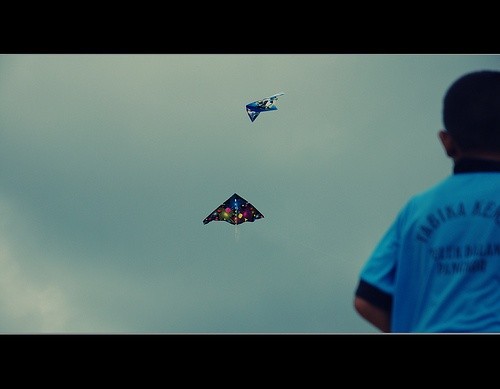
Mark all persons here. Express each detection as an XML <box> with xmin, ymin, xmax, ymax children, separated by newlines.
<box><xmin>352</xmin><ymin>69</ymin><xmax>499</xmax><ymax>333</ymax></box>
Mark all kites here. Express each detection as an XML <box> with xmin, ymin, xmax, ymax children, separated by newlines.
<box><xmin>202</xmin><ymin>192</ymin><xmax>265</xmax><ymax>227</ymax></box>
<box><xmin>244</xmin><ymin>93</ymin><xmax>285</xmax><ymax>123</ymax></box>
